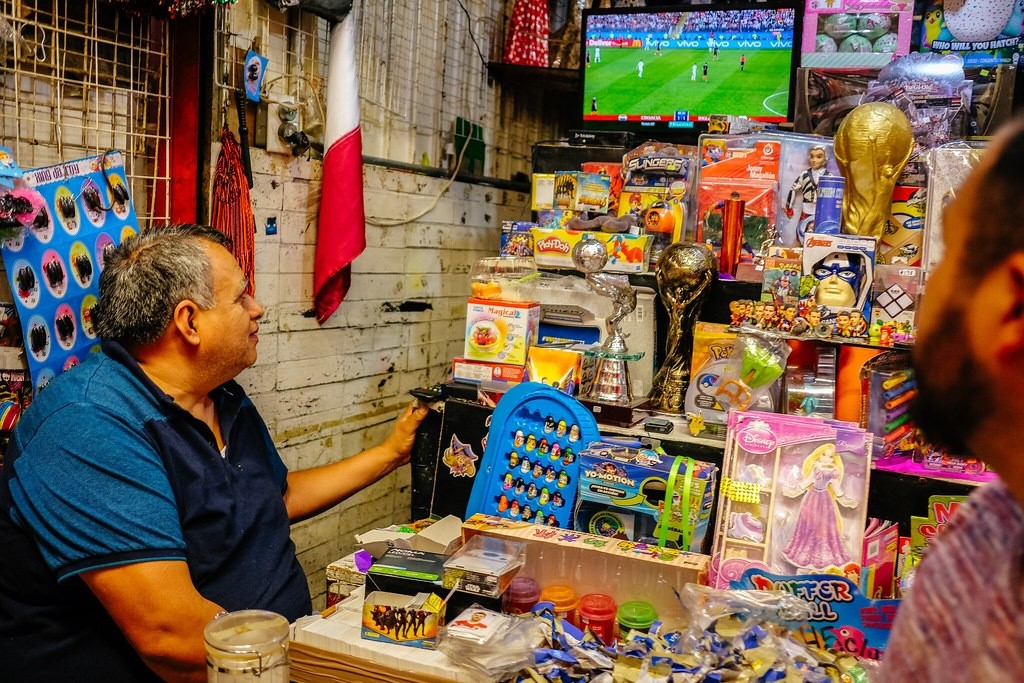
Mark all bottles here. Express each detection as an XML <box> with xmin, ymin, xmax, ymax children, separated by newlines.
<box><xmin>720</xmin><ymin>192</ymin><xmax>745</xmax><ymax>278</ymax></box>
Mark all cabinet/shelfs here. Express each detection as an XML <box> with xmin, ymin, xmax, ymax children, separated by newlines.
<box><xmin>410</xmin><ymin>257</ymin><xmax>998</xmax><ymax>547</ymax></box>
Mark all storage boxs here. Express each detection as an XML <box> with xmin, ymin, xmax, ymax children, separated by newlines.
<box><xmin>452</xmin><ymin>357</ymin><xmax>525</xmax><ymax>385</ymax></box>
<box><xmin>800</xmin><ymin>0</ymin><xmax>915</xmax><ymax>67</ymax></box>
<box><xmin>803</xmin><ymin>229</ymin><xmax>876</xmax><ymax>336</ymax></box>
<box><xmin>532</xmin><ymin>172</ymin><xmax>610</xmax><ymax>211</ymax></box>
<box><xmin>289</xmin><ymin>513</ymin><xmax>711</xmax><ymax>683</ymax></box>
<box><xmin>552</xmin><ymin>169</ymin><xmax>612</xmax><ymax>214</ymax></box>
<box><xmin>465</xmin><ymin>298</ymin><xmax>540</xmax><ymax>364</ymax></box>
<box><xmin>573</xmin><ymin>438</ymin><xmax>719</xmax><ymax>555</ymax></box>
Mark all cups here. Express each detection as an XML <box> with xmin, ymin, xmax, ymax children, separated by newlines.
<box><xmin>203</xmin><ymin>609</ymin><xmax>289</xmax><ymax>683</ymax></box>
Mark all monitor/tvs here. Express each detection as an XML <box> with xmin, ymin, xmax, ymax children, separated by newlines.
<box><xmin>580</xmin><ymin>0</ymin><xmax>805</xmax><ymax>145</ymax></box>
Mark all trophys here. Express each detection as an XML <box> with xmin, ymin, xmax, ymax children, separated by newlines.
<box><xmin>647</xmin><ymin>242</ymin><xmax>717</xmax><ymax>413</ymax></box>
<box><xmin>572</xmin><ymin>239</ymin><xmax>641</xmax><ymax>406</ymax></box>
<box><xmin>831</xmin><ymin>101</ymin><xmax>915</xmax><ymax>267</ymax></box>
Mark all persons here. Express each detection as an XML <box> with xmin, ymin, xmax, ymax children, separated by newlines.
<box><xmin>813</xmin><ymin>252</ymin><xmax>867</xmax><ymax>307</ymax></box>
<box><xmin>729</xmin><ymin>299</ymin><xmax>917</xmax><ymax>347</ymax></box>
<box><xmin>0</xmin><ymin>223</ymin><xmax>428</xmax><ymax>683</ymax></box>
<box><xmin>772</xmin><ymin>275</ymin><xmax>791</xmax><ymax>307</ymax></box>
<box><xmin>861</xmin><ymin>117</ymin><xmax>1024</xmax><ymax>682</ymax></box>
<box><xmin>780</xmin><ymin>143</ymin><xmax>834</xmax><ymax>248</ymax></box>
<box><xmin>582</xmin><ymin>8</ymin><xmax>795</xmax><ymax>116</ymax></box>
